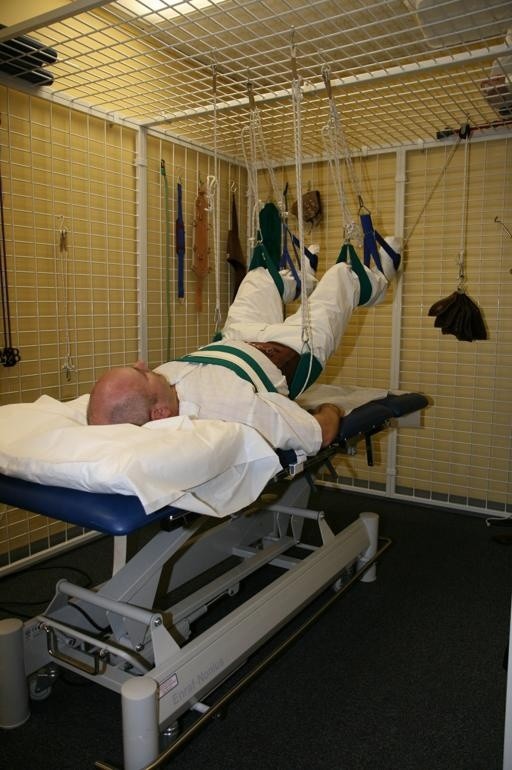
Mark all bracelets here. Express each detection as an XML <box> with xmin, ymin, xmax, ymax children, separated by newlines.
<box><xmin>318</xmin><ymin>403</ymin><xmax>345</xmax><ymax>418</ymax></box>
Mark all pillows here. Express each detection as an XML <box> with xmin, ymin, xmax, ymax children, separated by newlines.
<box><xmin>0</xmin><ymin>395</ymin><xmax>283</xmax><ymax>519</ymax></box>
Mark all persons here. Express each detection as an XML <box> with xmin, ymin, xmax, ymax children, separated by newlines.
<box><xmin>85</xmin><ymin>234</ymin><xmax>404</xmax><ymax>458</ymax></box>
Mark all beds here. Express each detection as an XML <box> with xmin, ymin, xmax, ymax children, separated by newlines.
<box><xmin>0</xmin><ymin>381</ymin><xmax>432</xmax><ymax>768</ymax></box>
